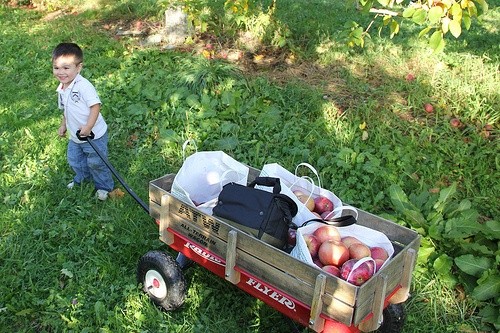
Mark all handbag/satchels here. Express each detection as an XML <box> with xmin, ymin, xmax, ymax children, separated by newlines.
<box><xmin>290</xmin><ymin>206</ymin><xmax>394</xmax><ymax>287</ymax></box>
<box><xmin>170</xmin><ymin>140</ymin><xmax>250</xmax><ymax>216</ymax></box>
<box><xmin>254</xmin><ymin>163</ymin><xmax>343</xmax><ymax>226</ymax></box>
<box><xmin>212</xmin><ymin>176</ymin><xmax>297</xmax><ymax>250</ymax></box>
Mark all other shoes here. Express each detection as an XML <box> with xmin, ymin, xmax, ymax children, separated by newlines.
<box><xmin>67</xmin><ymin>181</ymin><xmax>75</xmax><ymax>189</ymax></box>
<box><xmin>95</xmin><ymin>190</ymin><xmax>108</xmax><ymax>200</ymax></box>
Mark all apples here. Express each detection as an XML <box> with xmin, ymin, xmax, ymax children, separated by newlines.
<box><xmin>287</xmin><ymin>190</ymin><xmax>388</xmax><ymax>285</ymax></box>
<box><xmin>404</xmin><ymin>73</ymin><xmax>492</xmax><ymax>140</ymax></box>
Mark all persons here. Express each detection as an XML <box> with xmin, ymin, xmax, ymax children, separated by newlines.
<box><xmin>51</xmin><ymin>43</ymin><xmax>115</xmax><ymax>201</ymax></box>
